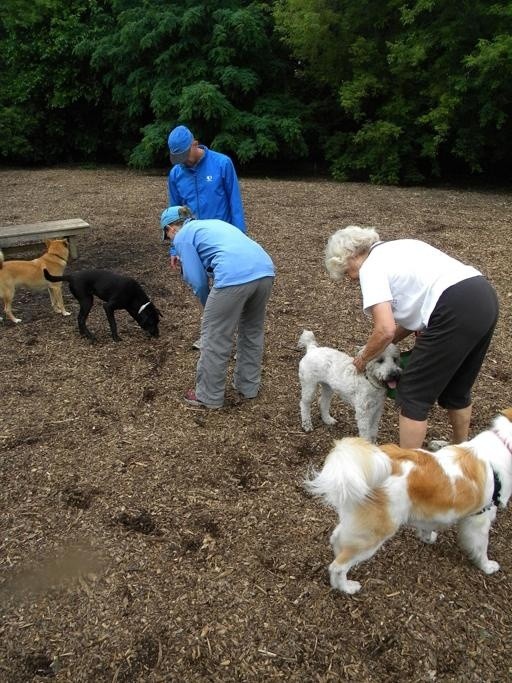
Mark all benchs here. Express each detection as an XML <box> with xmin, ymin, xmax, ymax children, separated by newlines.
<box><xmin>0</xmin><ymin>218</ymin><xmax>91</xmax><ymax>259</ymax></box>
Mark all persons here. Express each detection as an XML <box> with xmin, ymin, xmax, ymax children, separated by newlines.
<box><xmin>159</xmin><ymin>202</ymin><xmax>277</xmax><ymax>409</ymax></box>
<box><xmin>168</xmin><ymin>123</ymin><xmax>247</xmax><ymax>355</ymax></box>
<box><xmin>322</xmin><ymin>223</ymin><xmax>500</xmax><ymax>450</ymax></box>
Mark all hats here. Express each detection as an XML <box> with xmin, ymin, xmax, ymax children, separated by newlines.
<box><xmin>168</xmin><ymin>126</ymin><xmax>193</xmax><ymax>165</ymax></box>
<box><xmin>161</xmin><ymin>206</ymin><xmax>182</xmax><ymax>241</ymax></box>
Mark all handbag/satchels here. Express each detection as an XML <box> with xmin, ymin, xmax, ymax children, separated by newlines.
<box><xmin>387</xmin><ymin>349</ymin><xmax>413</xmax><ymax>399</ymax></box>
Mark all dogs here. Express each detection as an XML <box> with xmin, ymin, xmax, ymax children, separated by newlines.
<box><xmin>0</xmin><ymin>237</ymin><xmax>71</xmax><ymax>323</ymax></box>
<box><xmin>296</xmin><ymin>329</ymin><xmax>403</xmax><ymax>444</ymax></box>
<box><xmin>303</xmin><ymin>405</ymin><xmax>512</xmax><ymax>594</ymax></box>
<box><xmin>43</xmin><ymin>267</ymin><xmax>164</xmax><ymax>342</ymax></box>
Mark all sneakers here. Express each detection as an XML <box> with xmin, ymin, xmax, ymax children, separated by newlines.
<box><xmin>192</xmin><ymin>339</ymin><xmax>201</xmax><ymax>348</ymax></box>
<box><xmin>183</xmin><ymin>388</ymin><xmax>223</xmax><ymax>408</ymax></box>
